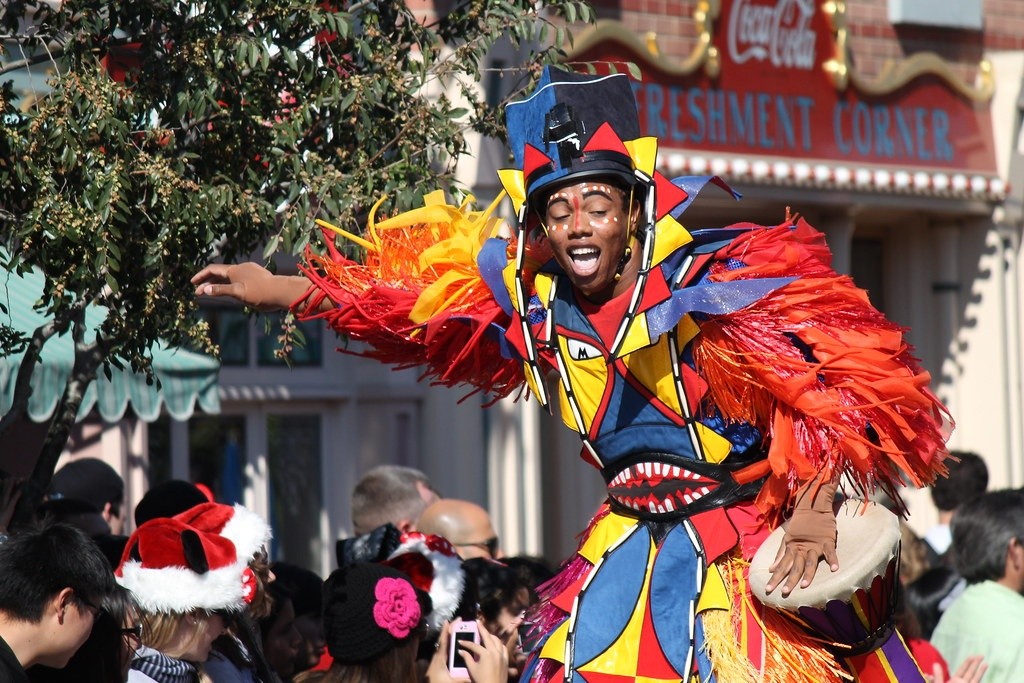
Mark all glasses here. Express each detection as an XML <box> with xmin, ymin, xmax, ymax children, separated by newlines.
<box><xmin>120</xmin><ymin>622</ymin><xmax>144</xmax><ymax>640</ymax></box>
<box><xmin>450</xmin><ymin>536</ymin><xmax>499</xmax><ymax>559</ymax></box>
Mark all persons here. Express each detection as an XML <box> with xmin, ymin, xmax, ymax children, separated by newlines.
<box><xmin>838</xmin><ymin>453</ymin><xmax>1024</xmax><ymax>683</ymax></box>
<box><xmin>0</xmin><ymin>458</ymin><xmax>564</xmax><ymax>683</ymax></box>
<box><xmin>191</xmin><ymin>176</ymin><xmax>950</xmax><ymax>683</ymax></box>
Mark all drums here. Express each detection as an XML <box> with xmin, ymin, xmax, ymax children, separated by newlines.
<box><xmin>747</xmin><ymin>496</ymin><xmax>904</xmax><ymax>658</ymax></box>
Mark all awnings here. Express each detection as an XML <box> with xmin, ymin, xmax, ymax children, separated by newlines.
<box><xmin>0</xmin><ymin>246</ymin><xmax>222</xmax><ymax>420</ymax></box>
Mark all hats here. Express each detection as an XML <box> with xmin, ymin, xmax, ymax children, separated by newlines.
<box><xmin>135</xmin><ymin>479</ymin><xmax>274</xmax><ymax>566</ymax></box>
<box><xmin>318</xmin><ymin>563</ymin><xmax>433</xmax><ymax>664</ymax></box>
<box><xmin>114</xmin><ymin>517</ymin><xmax>257</xmax><ymax>620</ymax></box>
<box><xmin>381</xmin><ymin>531</ymin><xmax>466</xmax><ymax>639</ymax></box>
<box><xmin>336</xmin><ymin>522</ymin><xmax>402</xmax><ymax>567</ymax></box>
<box><xmin>496</xmin><ymin>63</ymin><xmax>649</xmax><ymax>214</ymax></box>
<box><xmin>41</xmin><ymin>458</ymin><xmax>123</xmax><ymax>510</ymax></box>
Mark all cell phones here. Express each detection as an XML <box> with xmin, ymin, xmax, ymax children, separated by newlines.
<box><xmin>449</xmin><ymin>621</ymin><xmax>481</xmax><ymax>678</ymax></box>
